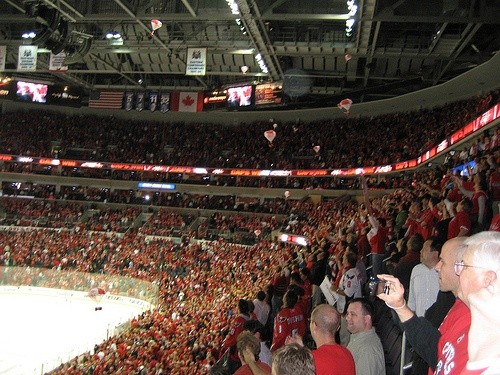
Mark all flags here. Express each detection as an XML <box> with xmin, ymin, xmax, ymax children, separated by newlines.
<box><xmin>170</xmin><ymin>91</ymin><xmax>205</xmax><ymax>113</ymax></box>
<box><xmin>88</xmin><ymin>90</ymin><xmax>124</xmax><ymax>108</ymax></box>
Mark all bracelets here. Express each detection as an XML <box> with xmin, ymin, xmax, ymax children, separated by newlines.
<box><xmin>385</xmin><ymin>299</ymin><xmax>406</xmax><ymax>309</ymax></box>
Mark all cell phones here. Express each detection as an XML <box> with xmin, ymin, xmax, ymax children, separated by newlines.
<box><xmin>292</xmin><ymin>329</ymin><xmax>298</xmax><ymax>338</ymax></box>
<box><xmin>384</xmin><ymin>281</ymin><xmax>390</xmax><ymax>295</ymax></box>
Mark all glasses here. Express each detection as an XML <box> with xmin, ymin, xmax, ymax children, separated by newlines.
<box><xmin>453</xmin><ymin>259</ymin><xmax>484</xmax><ymax>277</ymax></box>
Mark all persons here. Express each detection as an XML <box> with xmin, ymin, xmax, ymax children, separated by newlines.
<box><xmin>0</xmin><ymin>88</ymin><xmax>500</xmax><ymax>375</ymax></box>
